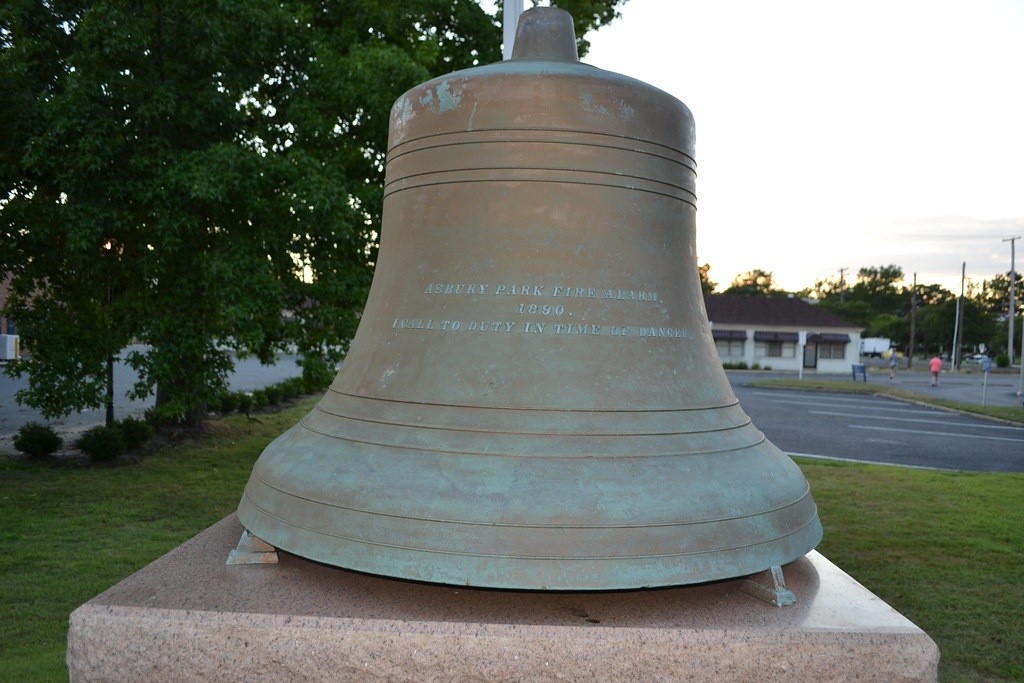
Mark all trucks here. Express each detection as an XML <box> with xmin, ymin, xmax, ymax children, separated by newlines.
<box><xmin>861</xmin><ymin>337</ymin><xmax>892</xmax><ymax>358</ymax></box>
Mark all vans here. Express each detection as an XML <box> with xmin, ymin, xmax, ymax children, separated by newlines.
<box><xmin>965</xmin><ymin>354</ymin><xmax>987</xmax><ymax>364</ymax></box>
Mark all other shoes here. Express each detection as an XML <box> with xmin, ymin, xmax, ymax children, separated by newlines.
<box><xmin>890</xmin><ymin>376</ymin><xmax>893</xmax><ymax>379</ymax></box>
<box><xmin>932</xmin><ymin>384</ymin><xmax>937</xmax><ymax>387</ymax></box>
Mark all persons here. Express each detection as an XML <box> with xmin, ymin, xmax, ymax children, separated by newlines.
<box><xmin>888</xmin><ymin>348</ymin><xmax>899</xmax><ymax>381</ymax></box>
<box><xmin>929</xmin><ymin>353</ymin><xmax>941</xmax><ymax>388</ymax></box>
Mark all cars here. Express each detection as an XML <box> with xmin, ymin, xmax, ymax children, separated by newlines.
<box><xmin>881</xmin><ymin>348</ymin><xmax>903</xmax><ymax>360</ymax></box>
